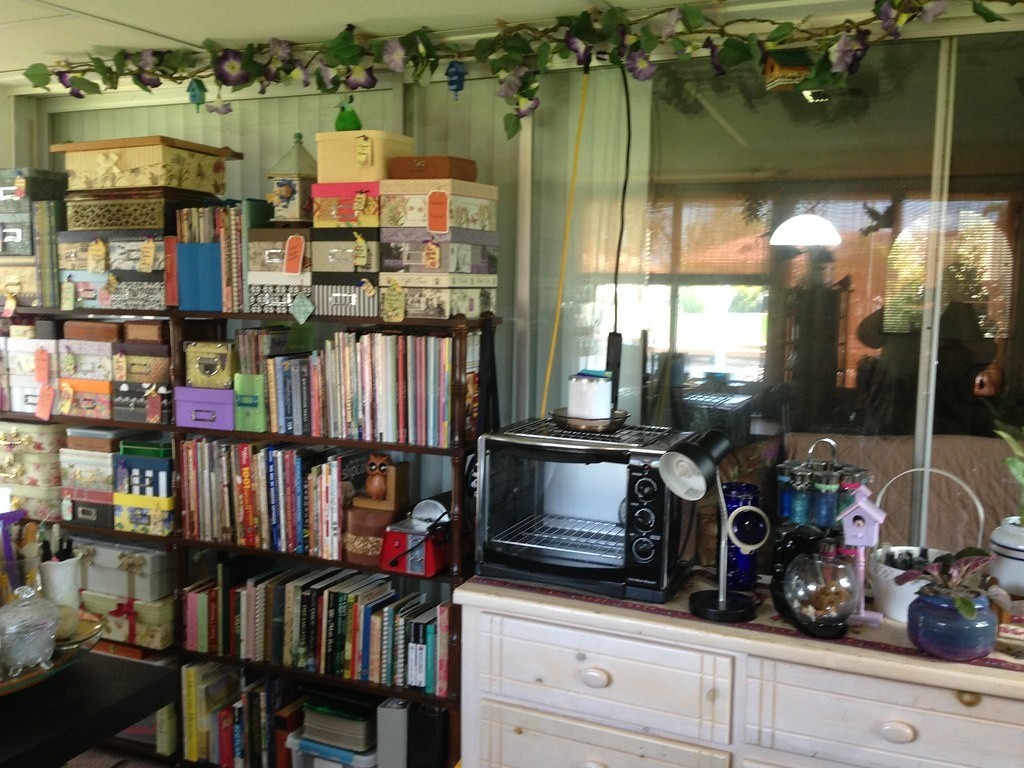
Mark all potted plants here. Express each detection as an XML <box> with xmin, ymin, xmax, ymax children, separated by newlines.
<box><xmin>989</xmin><ymin>428</ymin><xmax>1024</xmax><ymax>597</ymax></box>
<box><xmin>895</xmin><ymin>549</ymin><xmax>1000</xmax><ymax>660</ymax></box>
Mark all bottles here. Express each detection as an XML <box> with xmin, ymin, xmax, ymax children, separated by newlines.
<box><xmin>60</xmin><ymin>494</ymin><xmax>73</xmax><ymax>521</ymax></box>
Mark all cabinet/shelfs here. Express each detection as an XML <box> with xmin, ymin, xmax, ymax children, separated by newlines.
<box><xmin>739</xmin><ymin>651</ymin><xmax>1024</xmax><ymax>768</ymax></box>
<box><xmin>459</xmin><ymin>606</ymin><xmax>739</xmax><ymax>768</ymax></box>
<box><xmin>0</xmin><ymin>306</ymin><xmax>501</xmax><ymax>768</ymax></box>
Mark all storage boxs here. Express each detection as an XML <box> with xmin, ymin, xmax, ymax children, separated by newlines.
<box><xmin>0</xmin><ymin>320</ymin><xmax>170</xmax><ymax>651</ymax></box>
<box><xmin>184</xmin><ymin>340</ymin><xmax>239</xmax><ymax>390</ymax></box>
<box><xmin>315</xmin><ymin>130</ymin><xmax>414</xmax><ymax>183</ymax></box>
<box><xmin>310</xmin><ymin>228</ymin><xmax>380</xmax><ymax>287</ymax></box>
<box><xmin>247</xmin><ymin>227</ymin><xmax>311</xmax><ymax>273</ymax></box>
<box><xmin>65</xmin><ymin>145</ymin><xmax>225</xmax><ymax>195</ymax></box>
<box><xmin>0</xmin><ymin>168</ymin><xmax>68</xmax><ymax>307</ymax></box>
<box><xmin>284</xmin><ymin>726</ymin><xmax>378</xmax><ymax>768</ymax></box>
<box><xmin>379</xmin><ymin>179</ymin><xmax>498</xmax><ymax>246</ymax></box>
<box><xmin>389</xmin><ymin>156</ymin><xmax>478</xmax><ymax>182</ymax></box>
<box><xmin>311</xmin><ymin>285</ymin><xmax>378</xmax><ymax>317</ymax></box>
<box><xmin>471</xmin><ymin>245</ymin><xmax>488</xmax><ymax>274</ymax></box>
<box><xmin>65</xmin><ymin>198</ymin><xmax>236</xmax><ymax>231</ymax></box>
<box><xmin>59</xmin><ymin>269</ymin><xmax>165</xmax><ymax>311</ymax></box>
<box><xmin>56</xmin><ymin>230</ymin><xmax>164</xmax><ymax>271</ymax></box>
<box><xmin>410</xmin><ymin>243</ymin><xmax>471</xmax><ymax>275</ymax></box>
<box><xmin>248</xmin><ymin>271</ymin><xmax>312</xmax><ymax>315</ymax></box>
<box><xmin>311</xmin><ymin>181</ymin><xmax>380</xmax><ymax>227</ymax></box>
<box><xmin>378</xmin><ymin>272</ymin><xmax>498</xmax><ymax>319</ymax></box>
<box><xmin>173</xmin><ymin>386</ymin><xmax>234</xmax><ymax>431</ymax></box>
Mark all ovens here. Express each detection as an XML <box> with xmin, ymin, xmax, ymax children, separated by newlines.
<box><xmin>474</xmin><ymin>417</ymin><xmax>699</xmax><ymax>603</ymax></box>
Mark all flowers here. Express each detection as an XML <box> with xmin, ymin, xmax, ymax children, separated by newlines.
<box><xmin>24</xmin><ymin>0</ymin><xmax>1007</xmax><ymax>118</ymax></box>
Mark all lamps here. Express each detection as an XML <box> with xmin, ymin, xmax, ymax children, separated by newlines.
<box><xmin>660</xmin><ymin>430</ymin><xmax>758</xmax><ymax>624</ymax></box>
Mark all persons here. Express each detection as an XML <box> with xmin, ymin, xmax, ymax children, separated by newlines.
<box><xmin>856</xmin><ymin>302</ymin><xmax>997</xmax><ymax>438</ymax></box>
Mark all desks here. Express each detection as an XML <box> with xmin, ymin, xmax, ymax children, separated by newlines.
<box><xmin>0</xmin><ymin>647</ymin><xmax>175</xmax><ymax>768</ymax></box>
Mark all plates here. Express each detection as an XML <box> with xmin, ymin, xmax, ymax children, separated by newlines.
<box><xmin>55</xmin><ymin>619</ymin><xmax>103</xmax><ymax>650</ymax></box>
<box><xmin>550</xmin><ymin>408</ymin><xmax>631</xmax><ymax>433</ymax></box>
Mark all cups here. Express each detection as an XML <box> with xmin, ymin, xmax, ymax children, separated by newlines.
<box><xmin>718</xmin><ymin>481</ymin><xmax>762</xmax><ymax>591</ymax></box>
<box><xmin>0</xmin><ymin>554</ymin><xmax>38</xmax><ymax>606</ymax></box>
<box><xmin>567</xmin><ymin>377</ymin><xmax>612</xmax><ymax>421</ymax></box>
<box><xmin>39</xmin><ymin>549</ymin><xmax>83</xmax><ymax>611</ymax></box>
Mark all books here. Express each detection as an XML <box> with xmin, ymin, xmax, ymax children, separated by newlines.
<box><xmin>175</xmin><ymin>332</ymin><xmax>486</xmax><ymax>768</ymax></box>
